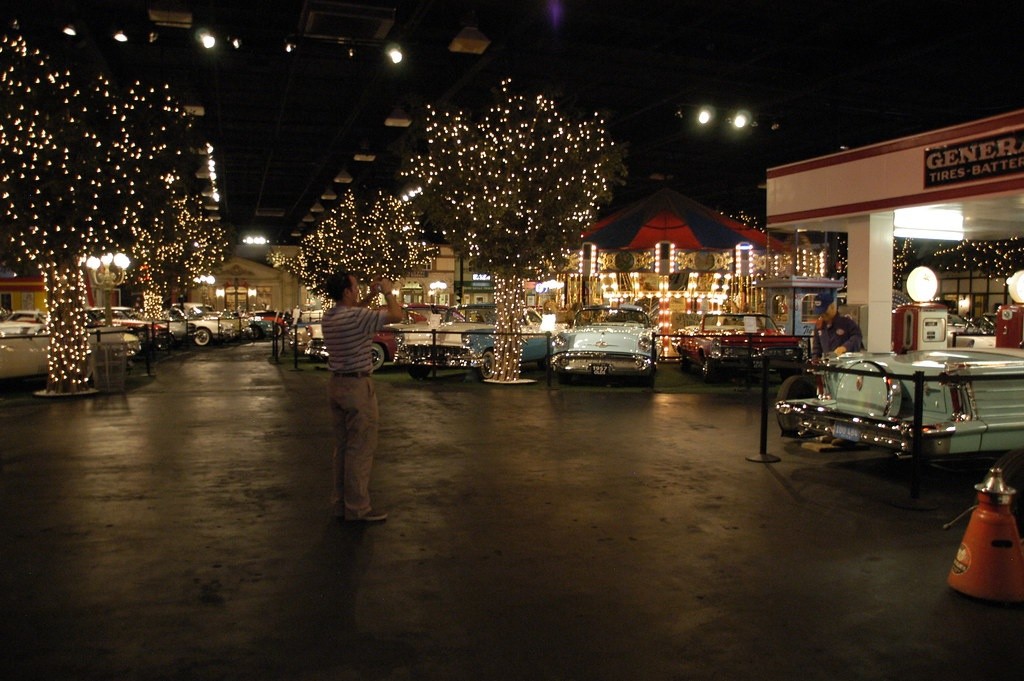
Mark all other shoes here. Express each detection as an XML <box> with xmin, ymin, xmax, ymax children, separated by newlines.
<box><xmin>330</xmin><ymin>513</ymin><xmax>387</xmax><ymax>522</ymax></box>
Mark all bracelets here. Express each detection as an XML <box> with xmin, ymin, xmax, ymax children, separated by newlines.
<box><xmin>385</xmin><ymin>292</ymin><xmax>391</xmax><ymax>298</ymax></box>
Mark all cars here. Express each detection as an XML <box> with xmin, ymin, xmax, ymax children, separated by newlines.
<box><xmin>0</xmin><ymin>302</ymin><xmax>287</xmax><ymax>379</ymax></box>
<box><xmin>551</xmin><ymin>304</ymin><xmax>657</xmax><ymax>388</ymax></box>
<box><xmin>677</xmin><ymin>313</ymin><xmax>804</xmax><ymax>384</ymax></box>
<box><xmin>396</xmin><ymin>303</ymin><xmax>551</xmax><ymax>381</ymax></box>
<box><xmin>304</xmin><ymin>304</ymin><xmax>450</xmax><ymax>369</ymax></box>
<box><xmin>776</xmin><ymin>349</ymin><xmax>1024</xmax><ymax>476</ymax></box>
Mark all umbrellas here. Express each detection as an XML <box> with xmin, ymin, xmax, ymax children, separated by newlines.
<box><xmin>576</xmin><ymin>186</ymin><xmax>794</xmax><ymax>356</ymax></box>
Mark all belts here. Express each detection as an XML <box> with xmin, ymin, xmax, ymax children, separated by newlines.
<box><xmin>335</xmin><ymin>370</ymin><xmax>372</xmax><ymax>378</ymax></box>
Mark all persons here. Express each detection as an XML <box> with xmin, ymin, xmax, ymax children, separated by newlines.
<box><xmin>322</xmin><ymin>271</ymin><xmax>403</xmax><ymax>521</ymax></box>
<box><xmin>277</xmin><ymin>312</ymin><xmax>293</xmax><ymax>341</ymax></box>
<box><xmin>811</xmin><ymin>292</ymin><xmax>865</xmax><ymax>356</ymax></box>
<box><xmin>284</xmin><ymin>312</ymin><xmax>294</xmax><ymax>327</ymax></box>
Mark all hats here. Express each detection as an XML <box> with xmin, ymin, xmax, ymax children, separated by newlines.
<box><xmin>813</xmin><ymin>293</ymin><xmax>834</xmax><ymax>314</ymax></box>
